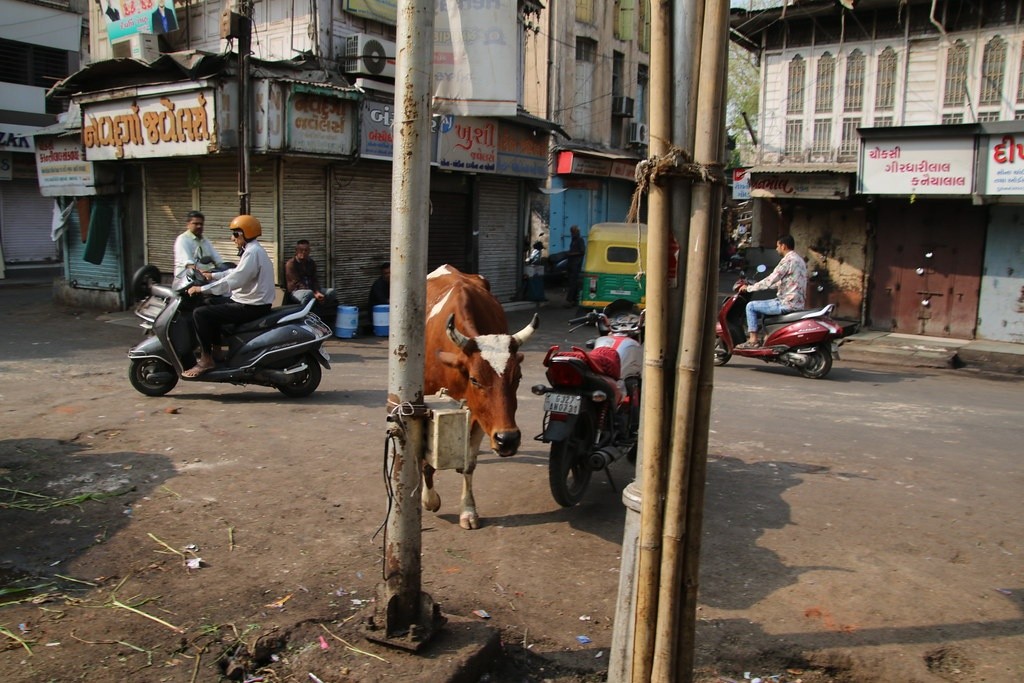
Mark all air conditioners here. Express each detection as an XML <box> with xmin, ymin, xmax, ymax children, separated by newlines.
<box><xmin>109</xmin><ymin>33</ymin><xmax>160</xmax><ymax>63</ymax></box>
<box><xmin>628</xmin><ymin>122</ymin><xmax>651</xmax><ymax>147</ymax></box>
<box><xmin>343</xmin><ymin>33</ymin><xmax>396</xmax><ymax>78</ymax></box>
<box><xmin>612</xmin><ymin>95</ymin><xmax>634</xmax><ymax>119</ymax></box>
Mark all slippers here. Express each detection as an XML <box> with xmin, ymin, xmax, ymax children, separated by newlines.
<box><xmin>181</xmin><ymin>361</ymin><xmax>215</xmax><ymax>378</ymax></box>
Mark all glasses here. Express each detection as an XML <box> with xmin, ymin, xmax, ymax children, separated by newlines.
<box><xmin>233</xmin><ymin>232</ymin><xmax>243</xmax><ymax>238</ymax></box>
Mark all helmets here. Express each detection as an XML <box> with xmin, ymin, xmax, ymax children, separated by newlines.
<box><xmin>229</xmin><ymin>215</ymin><xmax>261</xmax><ymax>240</ymax></box>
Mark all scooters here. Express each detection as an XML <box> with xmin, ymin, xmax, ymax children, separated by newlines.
<box><xmin>713</xmin><ymin>264</ymin><xmax>845</xmax><ymax>378</ymax></box>
<box><xmin>126</xmin><ymin>246</ymin><xmax>333</xmax><ymax>398</ymax></box>
<box><xmin>523</xmin><ymin>232</ymin><xmax>570</xmax><ymax>286</ymax></box>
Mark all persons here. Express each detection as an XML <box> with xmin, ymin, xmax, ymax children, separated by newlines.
<box><xmin>368</xmin><ymin>262</ymin><xmax>390</xmax><ymax>317</ymax></box>
<box><xmin>564</xmin><ymin>225</ymin><xmax>586</xmax><ymax>301</ymax></box>
<box><xmin>99</xmin><ymin>0</ymin><xmax>120</xmax><ymax>23</ymax></box>
<box><xmin>173</xmin><ymin>211</ymin><xmax>222</xmax><ymax>290</ymax></box>
<box><xmin>181</xmin><ymin>215</ymin><xmax>274</xmax><ymax>377</ymax></box>
<box><xmin>286</xmin><ymin>239</ymin><xmax>338</xmax><ymax>327</ymax></box>
<box><xmin>735</xmin><ymin>234</ymin><xmax>806</xmax><ymax>349</ymax></box>
<box><xmin>151</xmin><ymin>0</ymin><xmax>178</xmax><ymax>37</ymax></box>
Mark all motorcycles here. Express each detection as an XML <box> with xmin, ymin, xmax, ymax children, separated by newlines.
<box><xmin>532</xmin><ymin>298</ymin><xmax>646</xmax><ymax>507</ymax></box>
<box><xmin>577</xmin><ymin>222</ymin><xmax>648</xmax><ymax>310</ymax></box>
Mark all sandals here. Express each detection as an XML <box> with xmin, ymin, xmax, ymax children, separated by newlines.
<box><xmin>736</xmin><ymin>341</ymin><xmax>760</xmax><ymax>349</ymax></box>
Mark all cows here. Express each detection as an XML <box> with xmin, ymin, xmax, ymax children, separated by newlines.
<box><xmin>423</xmin><ymin>264</ymin><xmax>540</xmax><ymax>532</ymax></box>
